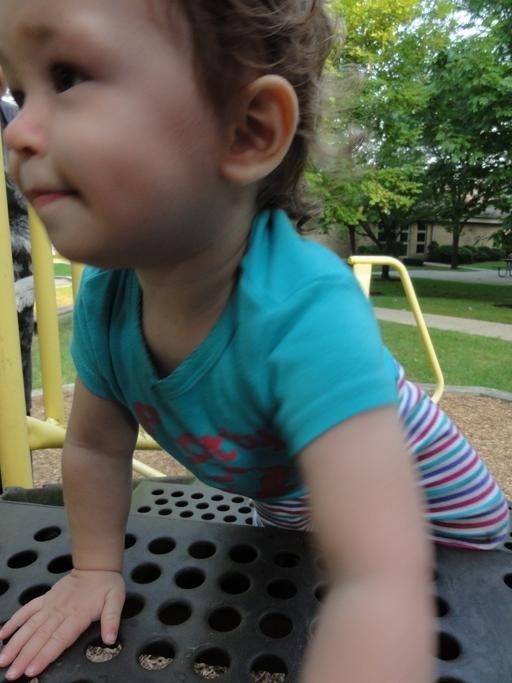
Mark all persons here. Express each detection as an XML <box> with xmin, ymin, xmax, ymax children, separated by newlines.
<box><xmin>1</xmin><ymin>0</ymin><xmax>511</xmax><ymax>678</ymax></box>
<box><xmin>0</xmin><ymin>65</ymin><xmax>38</xmax><ymax>424</ymax></box>
